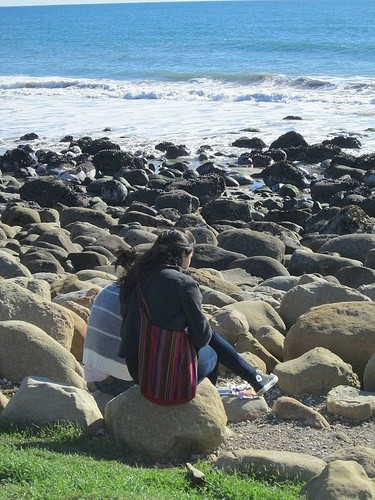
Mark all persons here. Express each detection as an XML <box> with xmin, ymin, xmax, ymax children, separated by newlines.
<box><xmin>116</xmin><ymin>225</ymin><xmax>280</xmax><ymax>408</ymax></box>
<box><xmin>81</xmin><ymin>244</ymin><xmax>140</xmax><ymax>395</ymax></box>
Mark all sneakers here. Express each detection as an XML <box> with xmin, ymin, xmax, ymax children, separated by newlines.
<box><xmin>250</xmin><ymin>369</ymin><xmax>278</xmax><ymax>396</ymax></box>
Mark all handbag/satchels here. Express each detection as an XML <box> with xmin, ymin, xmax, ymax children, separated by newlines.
<box><xmin>135</xmin><ymin>279</ymin><xmax>198</xmax><ymax>405</ymax></box>
